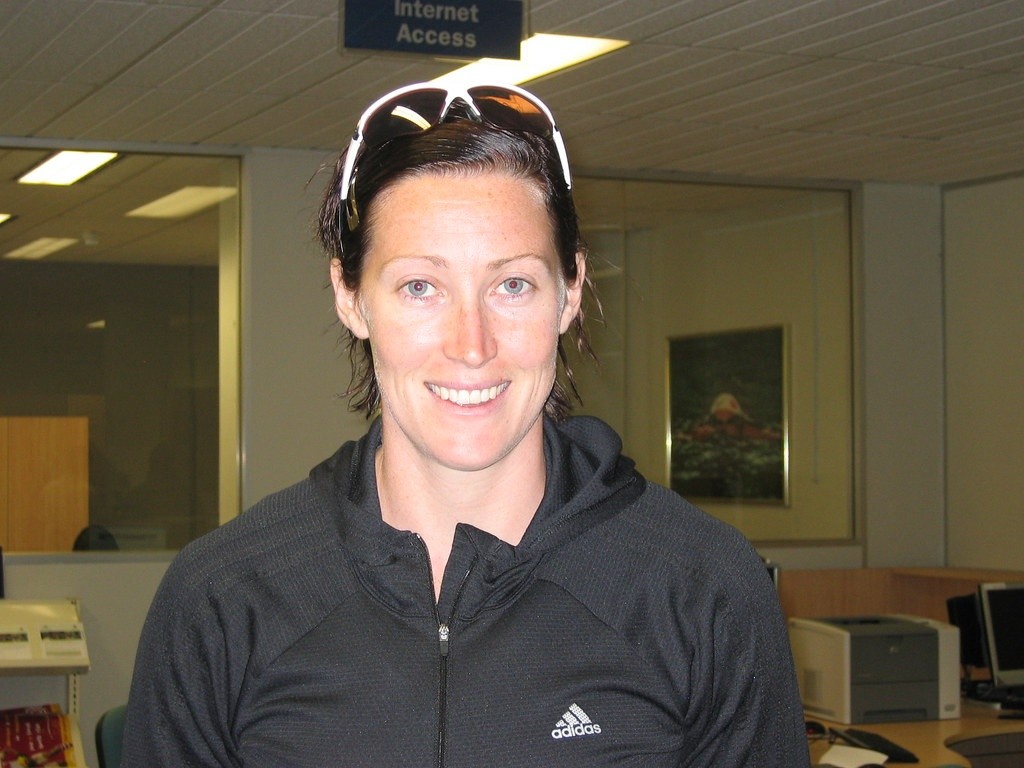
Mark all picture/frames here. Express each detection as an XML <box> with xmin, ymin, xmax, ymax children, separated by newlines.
<box><xmin>661</xmin><ymin>322</ymin><xmax>792</xmax><ymax>510</ymax></box>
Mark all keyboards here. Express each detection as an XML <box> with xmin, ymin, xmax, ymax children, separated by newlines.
<box><xmin>828</xmin><ymin>727</ymin><xmax>920</xmax><ymax>764</ymax></box>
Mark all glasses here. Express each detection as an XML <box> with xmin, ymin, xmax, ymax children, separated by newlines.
<box><xmin>338</xmin><ymin>80</ymin><xmax>573</xmax><ymax>202</ymax></box>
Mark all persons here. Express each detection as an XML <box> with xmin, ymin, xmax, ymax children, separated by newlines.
<box><xmin>122</xmin><ymin>79</ymin><xmax>813</xmax><ymax>766</ymax></box>
<box><xmin>673</xmin><ymin>390</ymin><xmax>781</xmax><ymax>442</ymax></box>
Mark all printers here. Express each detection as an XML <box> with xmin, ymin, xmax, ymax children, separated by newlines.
<box><xmin>787</xmin><ymin>613</ymin><xmax>961</xmax><ymax>725</ymax></box>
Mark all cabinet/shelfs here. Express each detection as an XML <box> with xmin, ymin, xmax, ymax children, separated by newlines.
<box><xmin>0</xmin><ymin>415</ymin><xmax>90</xmax><ymax>552</ymax></box>
<box><xmin>0</xmin><ymin>599</ymin><xmax>91</xmax><ymax>768</ymax></box>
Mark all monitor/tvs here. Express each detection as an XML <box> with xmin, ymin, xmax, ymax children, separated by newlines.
<box><xmin>946</xmin><ymin>582</ymin><xmax>1024</xmax><ymax>710</ymax></box>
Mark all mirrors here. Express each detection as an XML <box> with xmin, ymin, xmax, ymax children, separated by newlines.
<box><xmin>0</xmin><ymin>135</ymin><xmax>254</xmax><ymax>568</ymax></box>
<box><xmin>557</xmin><ymin>164</ymin><xmax>868</xmax><ymax>548</ymax></box>
